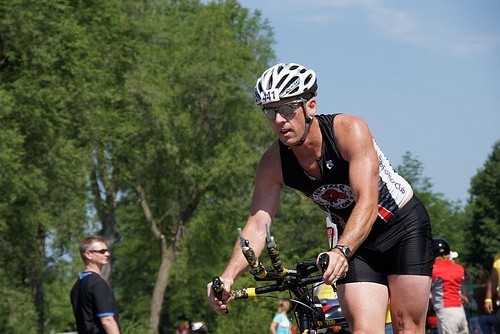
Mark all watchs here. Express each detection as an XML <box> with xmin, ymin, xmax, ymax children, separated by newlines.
<box><xmin>335</xmin><ymin>244</ymin><xmax>352</xmax><ymax>259</ymax></box>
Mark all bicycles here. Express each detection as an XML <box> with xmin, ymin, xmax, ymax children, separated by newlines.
<box><xmin>211</xmin><ymin>222</ymin><xmax>349</xmax><ymax>334</ymax></box>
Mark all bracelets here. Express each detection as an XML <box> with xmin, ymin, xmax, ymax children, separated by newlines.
<box><xmin>485</xmin><ymin>299</ymin><xmax>492</xmax><ymax>302</ymax></box>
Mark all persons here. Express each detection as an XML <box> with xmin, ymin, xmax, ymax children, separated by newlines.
<box><xmin>268</xmin><ymin>301</ymin><xmax>290</xmax><ymax>334</ymax></box>
<box><xmin>206</xmin><ymin>62</ymin><xmax>435</xmax><ymax>334</ymax></box>
<box><xmin>484</xmin><ymin>254</ymin><xmax>500</xmax><ymax>313</ymax></box>
<box><xmin>69</xmin><ymin>235</ymin><xmax>123</xmax><ymax>334</ymax></box>
<box><xmin>465</xmin><ymin>297</ymin><xmax>483</xmax><ymax>334</ymax></box>
<box><xmin>431</xmin><ymin>238</ymin><xmax>470</xmax><ymax>334</ymax></box>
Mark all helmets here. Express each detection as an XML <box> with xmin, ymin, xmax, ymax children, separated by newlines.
<box><xmin>253</xmin><ymin>63</ymin><xmax>318</xmax><ymax>106</ymax></box>
<box><xmin>434</xmin><ymin>238</ymin><xmax>450</xmax><ymax>256</ymax></box>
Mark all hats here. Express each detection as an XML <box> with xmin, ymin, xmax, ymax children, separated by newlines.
<box><xmin>449</xmin><ymin>250</ymin><xmax>458</xmax><ymax>260</ymax></box>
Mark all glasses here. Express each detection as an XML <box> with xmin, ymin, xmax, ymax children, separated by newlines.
<box><xmin>85</xmin><ymin>249</ymin><xmax>107</xmax><ymax>254</ymax></box>
<box><xmin>262</xmin><ymin>99</ymin><xmax>307</xmax><ymax>120</ymax></box>
